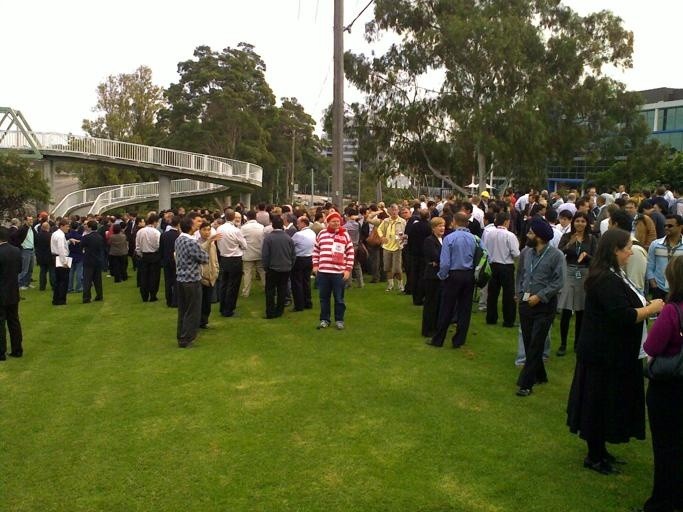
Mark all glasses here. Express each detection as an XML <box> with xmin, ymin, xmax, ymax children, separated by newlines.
<box><xmin>663</xmin><ymin>223</ymin><xmax>679</xmax><ymax>228</ymax></box>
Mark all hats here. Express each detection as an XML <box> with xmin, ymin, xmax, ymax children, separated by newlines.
<box><xmin>530</xmin><ymin>220</ymin><xmax>555</xmax><ymax>242</ymax></box>
<box><xmin>326</xmin><ymin>212</ymin><xmax>342</xmax><ymax>224</ymax></box>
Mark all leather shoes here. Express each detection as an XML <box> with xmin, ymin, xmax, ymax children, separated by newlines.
<box><xmin>175</xmin><ymin>310</ymin><xmax>313</xmax><ymax>348</ymax></box>
<box><xmin>485</xmin><ymin>319</ymin><xmax>499</xmax><ymax>325</ymax></box>
<box><xmin>502</xmin><ymin>323</ymin><xmax>520</xmax><ymax>328</ymax></box>
<box><xmin>5</xmin><ymin>349</ymin><xmax>24</xmax><ymax>358</ymax></box>
<box><xmin>515</xmin><ymin>387</ymin><xmax>533</xmax><ymax>397</ymax></box>
<box><xmin>582</xmin><ymin>455</ymin><xmax>627</xmax><ymax>477</ymax></box>
<box><xmin>426</xmin><ymin>337</ymin><xmax>445</xmax><ymax>348</ymax></box>
<box><xmin>450</xmin><ymin>342</ymin><xmax>466</xmax><ymax>349</ymax></box>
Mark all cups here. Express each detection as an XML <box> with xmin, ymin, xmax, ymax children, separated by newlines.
<box><xmin>398</xmin><ymin>231</ymin><xmax>403</xmax><ymax>237</ymax></box>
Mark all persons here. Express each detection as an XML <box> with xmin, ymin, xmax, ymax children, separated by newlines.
<box><xmin>1</xmin><ymin>185</ymin><xmax>683</xmax><ymax>369</ymax></box>
<box><xmin>641</xmin><ymin>252</ymin><xmax>683</xmax><ymax>511</ymax></box>
<box><xmin>565</xmin><ymin>228</ymin><xmax>666</xmax><ymax>477</ymax></box>
<box><xmin>513</xmin><ymin>215</ymin><xmax>565</xmax><ymax>398</ymax></box>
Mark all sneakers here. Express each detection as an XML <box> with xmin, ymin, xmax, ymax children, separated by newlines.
<box><xmin>512</xmin><ymin>347</ymin><xmax>568</xmax><ymax>368</ymax></box>
<box><xmin>17</xmin><ymin>286</ymin><xmax>27</xmax><ymax>291</ymax></box>
<box><xmin>316</xmin><ymin>320</ymin><xmax>330</xmax><ymax>329</ymax></box>
<box><xmin>26</xmin><ymin>284</ymin><xmax>36</xmax><ymax>289</ymax></box>
<box><xmin>334</xmin><ymin>321</ymin><xmax>345</xmax><ymax>330</ymax></box>
<box><xmin>345</xmin><ymin>274</ymin><xmax>426</xmax><ymax>305</ymax></box>
<box><xmin>52</xmin><ymin>275</ymin><xmax>179</xmax><ymax>310</ymax></box>
